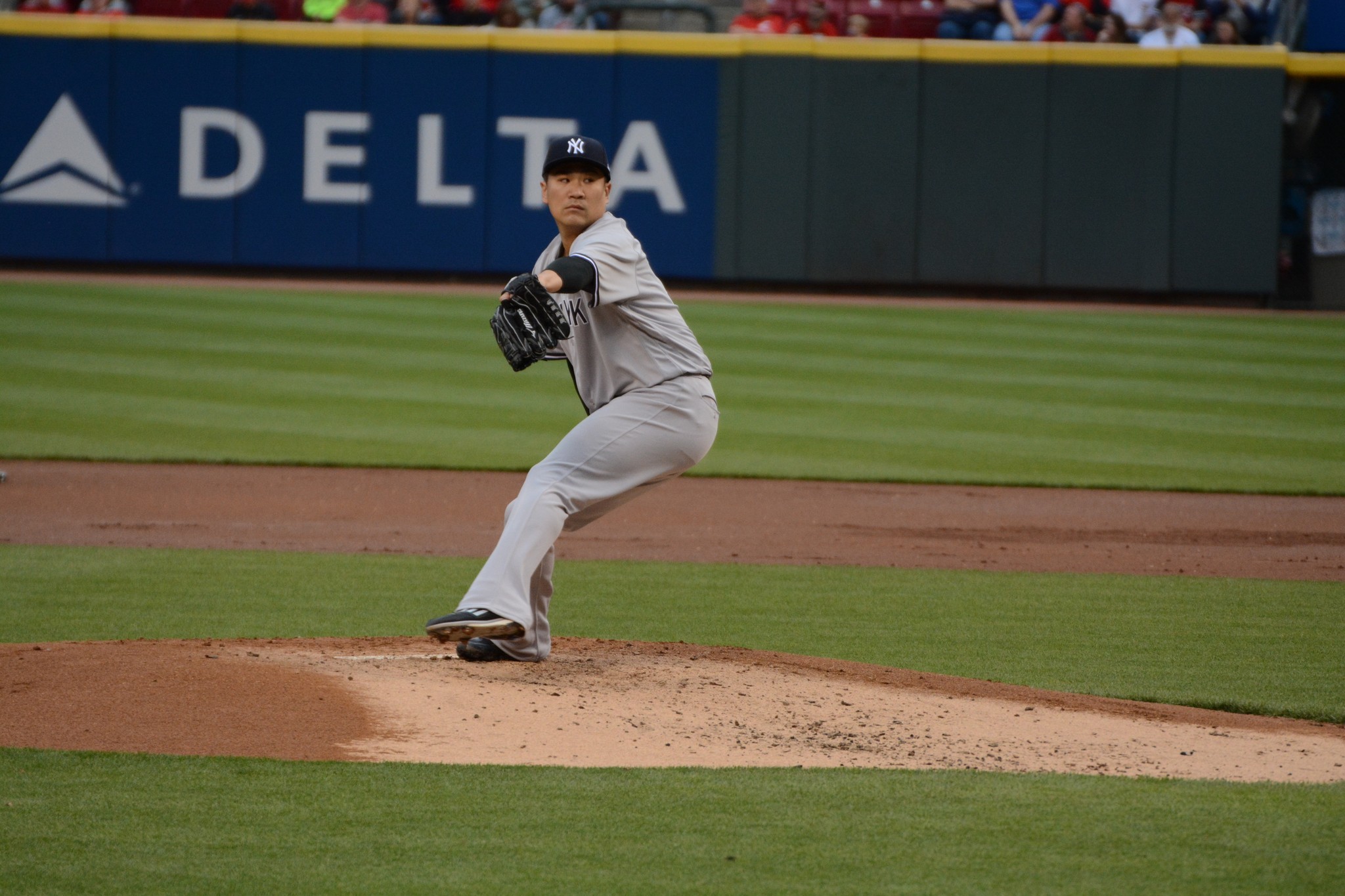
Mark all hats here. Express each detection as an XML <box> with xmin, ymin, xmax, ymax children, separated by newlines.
<box><xmin>541</xmin><ymin>136</ymin><xmax>612</xmax><ymax>185</ymax></box>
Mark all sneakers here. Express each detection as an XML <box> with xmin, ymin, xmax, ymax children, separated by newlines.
<box><xmin>455</xmin><ymin>636</ymin><xmax>511</xmax><ymax>663</ymax></box>
<box><xmin>426</xmin><ymin>607</ymin><xmax>523</xmax><ymax>645</ymax></box>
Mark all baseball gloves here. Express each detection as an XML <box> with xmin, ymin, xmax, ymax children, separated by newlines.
<box><xmin>489</xmin><ymin>274</ymin><xmax>571</xmax><ymax>373</ymax></box>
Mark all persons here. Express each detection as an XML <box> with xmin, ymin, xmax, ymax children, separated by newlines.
<box><xmin>0</xmin><ymin>0</ymin><xmax>1345</xmax><ymax>58</ymax></box>
<box><xmin>427</xmin><ymin>133</ymin><xmax>720</xmax><ymax>664</ymax></box>
